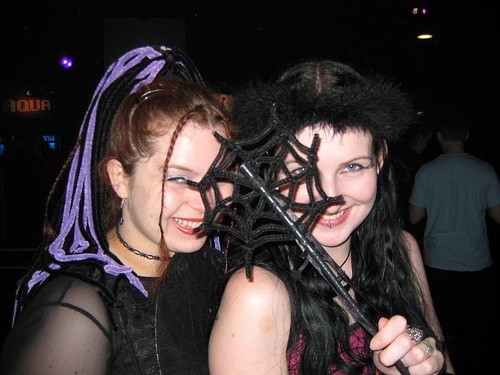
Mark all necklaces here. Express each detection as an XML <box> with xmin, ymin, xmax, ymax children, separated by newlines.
<box><xmin>114</xmin><ymin>222</ymin><xmax>174</xmax><ymax>261</ymax></box>
<box><xmin>324</xmin><ymin>248</ymin><xmax>350</xmax><ymax>282</ymax></box>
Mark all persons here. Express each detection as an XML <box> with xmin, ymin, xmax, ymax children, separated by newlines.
<box><xmin>1</xmin><ymin>44</ymin><xmax>240</xmax><ymax>374</ymax></box>
<box><xmin>207</xmin><ymin>62</ymin><xmax>456</xmax><ymax>375</ymax></box>
<box><xmin>385</xmin><ymin>114</ymin><xmax>499</xmax><ymax>357</ymax></box>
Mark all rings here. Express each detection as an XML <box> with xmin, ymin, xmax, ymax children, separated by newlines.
<box><xmin>405</xmin><ymin>327</ymin><xmax>423</xmax><ymax>344</ymax></box>
<box><xmin>420</xmin><ymin>341</ymin><xmax>434</xmax><ymax>358</ymax></box>
